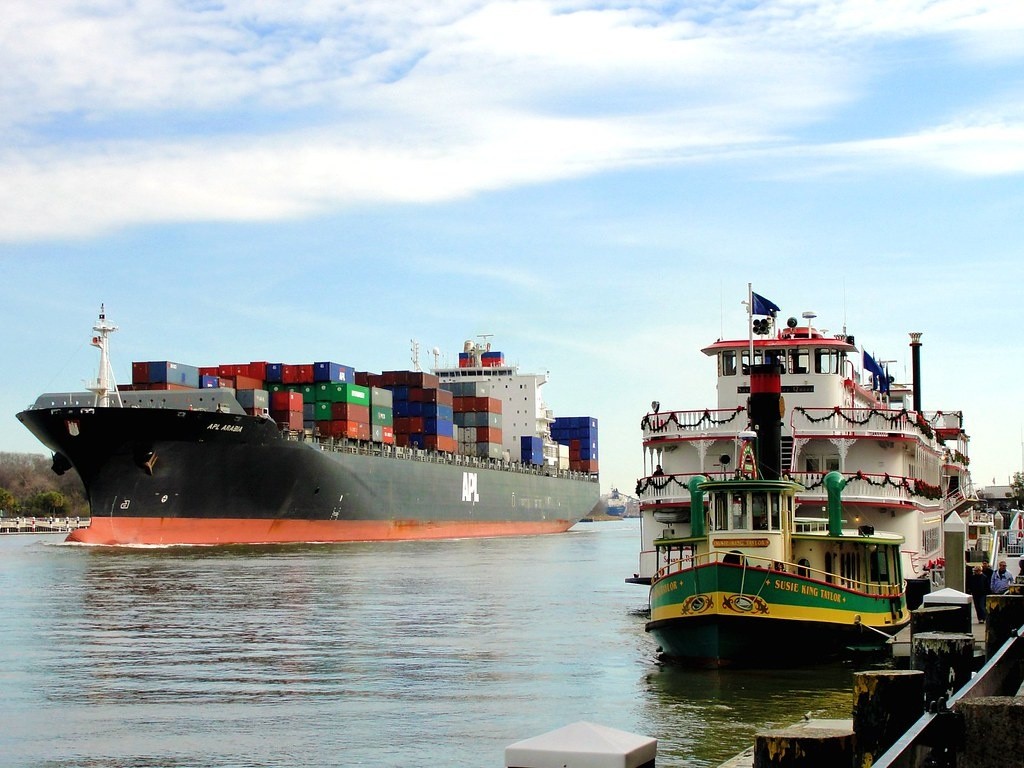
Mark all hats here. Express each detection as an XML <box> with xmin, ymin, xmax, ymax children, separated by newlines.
<box><xmin>973</xmin><ymin>565</ymin><xmax>982</xmax><ymax>570</ymax></box>
<box><xmin>1020</xmin><ymin>555</ymin><xmax>1024</xmax><ymax>560</ymax></box>
<box><xmin>999</xmin><ymin>561</ymin><xmax>1007</xmax><ymax>565</ymax></box>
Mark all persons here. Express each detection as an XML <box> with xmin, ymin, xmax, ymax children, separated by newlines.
<box><xmin>969</xmin><ymin>566</ymin><xmax>989</xmax><ymax>623</ymax></box>
<box><xmin>1016</xmin><ymin>560</ymin><xmax>1024</xmax><ymax>594</ymax></box>
<box><xmin>990</xmin><ymin>561</ymin><xmax>1014</xmax><ymax>594</ymax></box>
<box><xmin>981</xmin><ymin>560</ymin><xmax>994</xmax><ymax>582</ymax></box>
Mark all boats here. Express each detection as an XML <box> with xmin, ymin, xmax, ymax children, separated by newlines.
<box><xmin>605</xmin><ymin>487</ymin><xmax>627</xmax><ymax>518</ymax></box>
<box><xmin>625</xmin><ymin>283</ymin><xmax>995</xmax><ymax>675</ymax></box>
<box><xmin>15</xmin><ymin>303</ymin><xmax>601</xmax><ymax>547</ymax></box>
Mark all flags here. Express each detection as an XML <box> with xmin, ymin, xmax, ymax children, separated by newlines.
<box><xmin>752</xmin><ymin>291</ymin><xmax>780</xmax><ymax>316</ymax></box>
<box><xmin>864</xmin><ymin>351</ymin><xmax>891</xmax><ymax>396</ymax></box>
<box><xmin>987</xmin><ymin>530</ymin><xmax>1000</xmax><ymax>571</ymax></box>
<box><xmin>1008</xmin><ymin>512</ymin><xmax>1024</xmax><ymax>548</ymax></box>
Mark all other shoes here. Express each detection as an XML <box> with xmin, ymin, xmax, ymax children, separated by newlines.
<box><xmin>979</xmin><ymin>620</ymin><xmax>984</xmax><ymax>624</ymax></box>
